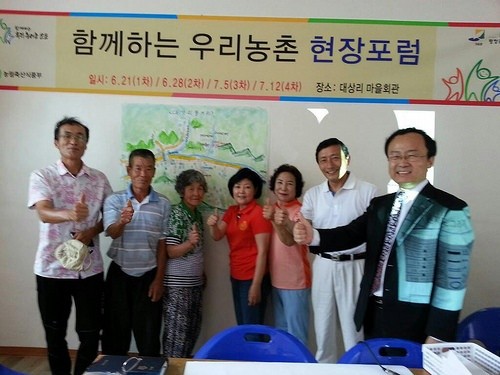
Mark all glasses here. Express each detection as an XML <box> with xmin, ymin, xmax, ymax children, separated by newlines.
<box><xmin>59</xmin><ymin>132</ymin><xmax>86</xmax><ymax>142</ymax></box>
<box><xmin>389</xmin><ymin>151</ymin><xmax>428</xmax><ymax>162</ymax></box>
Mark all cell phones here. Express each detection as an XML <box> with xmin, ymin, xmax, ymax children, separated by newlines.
<box><xmin>70</xmin><ymin>232</ymin><xmax>94</xmax><ymax>247</ymax></box>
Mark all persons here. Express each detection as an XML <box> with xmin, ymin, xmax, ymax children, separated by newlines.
<box><xmin>262</xmin><ymin>164</ymin><xmax>312</xmax><ymax>348</ymax></box>
<box><xmin>274</xmin><ymin>138</ymin><xmax>379</xmax><ymax>364</ymax></box>
<box><xmin>27</xmin><ymin>118</ymin><xmax>114</xmax><ymax>375</ymax></box>
<box><xmin>206</xmin><ymin>168</ymin><xmax>272</xmax><ymax>325</ymax></box>
<box><xmin>101</xmin><ymin>149</ymin><xmax>172</xmax><ymax>357</ymax></box>
<box><xmin>162</xmin><ymin>169</ymin><xmax>208</xmax><ymax>359</ymax></box>
<box><xmin>293</xmin><ymin>127</ymin><xmax>475</xmax><ymax>356</ymax></box>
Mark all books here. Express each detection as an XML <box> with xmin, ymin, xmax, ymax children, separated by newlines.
<box><xmin>81</xmin><ymin>356</ymin><xmax>169</xmax><ymax>375</ymax></box>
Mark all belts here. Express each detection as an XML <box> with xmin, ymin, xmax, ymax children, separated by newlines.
<box><xmin>316</xmin><ymin>252</ymin><xmax>367</xmax><ymax>261</ymax></box>
<box><xmin>369</xmin><ymin>297</ymin><xmax>384</xmax><ymax>310</ymax></box>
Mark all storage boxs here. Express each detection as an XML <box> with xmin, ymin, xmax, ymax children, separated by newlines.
<box><xmin>422</xmin><ymin>343</ymin><xmax>500</xmax><ymax>375</ymax></box>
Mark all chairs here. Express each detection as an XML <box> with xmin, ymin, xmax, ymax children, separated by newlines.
<box><xmin>193</xmin><ymin>307</ymin><xmax>500</xmax><ymax>368</ymax></box>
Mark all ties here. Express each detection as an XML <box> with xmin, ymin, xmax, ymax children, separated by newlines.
<box><xmin>370</xmin><ymin>190</ymin><xmax>405</xmax><ymax>293</ymax></box>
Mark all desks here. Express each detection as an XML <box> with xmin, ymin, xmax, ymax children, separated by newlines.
<box><xmin>81</xmin><ymin>355</ymin><xmax>430</xmax><ymax>375</ymax></box>
